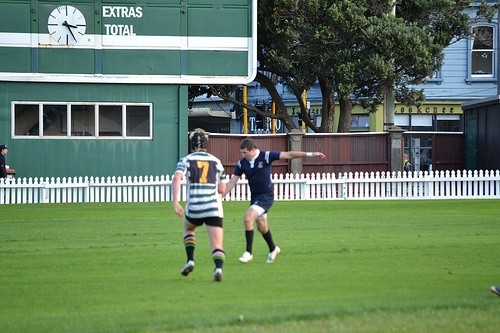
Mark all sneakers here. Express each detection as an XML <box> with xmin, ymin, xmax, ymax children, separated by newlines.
<box><xmin>214</xmin><ymin>268</ymin><xmax>222</xmax><ymax>281</ymax></box>
<box><xmin>182</xmin><ymin>261</ymin><xmax>194</xmax><ymax>275</ymax></box>
<box><xmin>265</xmin><ymin>246</ymin><xmax>281</xmax><ymax>263</ymax></box>
<box><xmin>238</xmin><ymin>251</ymin><xmax>253</xmax><ymax>262</ymax></box>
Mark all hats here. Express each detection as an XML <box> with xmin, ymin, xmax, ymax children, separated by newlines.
<box><xmin>0</xmin><ymin>145</ymin><xmax>8</xmax><ymax>150</ymax></box>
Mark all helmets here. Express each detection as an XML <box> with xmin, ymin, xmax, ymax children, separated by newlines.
<box><xmin>192</xmin><ymin>130</ymin><xmax>207</xmax><ymax>149</ymax></box>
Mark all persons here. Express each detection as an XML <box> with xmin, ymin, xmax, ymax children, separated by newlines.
<box><xmin>172</xmin><ymin>128</ymin><xmax>227</xmax><ymax>281</ymax></box>
<box><xmin>0</xmin><ymin>144</ymin><xmax>8</xmax><ymax>179</ymax></box>
<box><xmin>220</xmin><ymin>139</ymin><xmax>326</xmax><ymax>264</ymax></box>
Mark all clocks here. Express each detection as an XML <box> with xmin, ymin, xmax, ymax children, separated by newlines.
<box><xmin>47</xmin><ymin>5</ymin><xmax>87</xmax><ymax>46</ymax></box>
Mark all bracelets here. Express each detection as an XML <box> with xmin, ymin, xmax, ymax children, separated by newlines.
<box><xmin>307</xmin><ymin>153</ymin><xmax>312</xmax><ymax>158</ymax></box>
